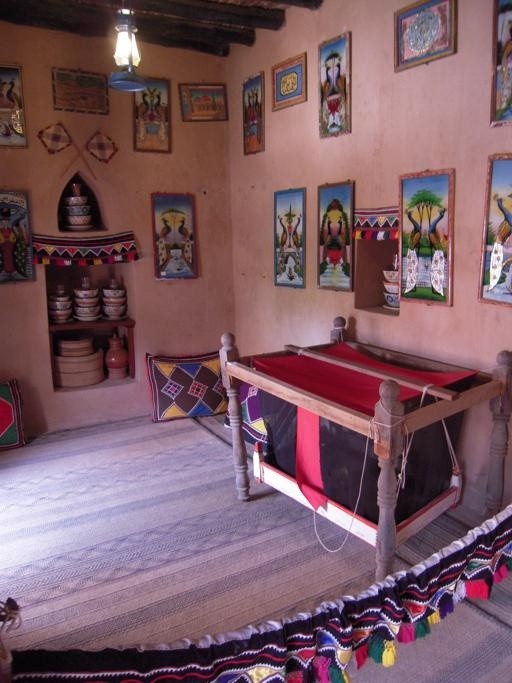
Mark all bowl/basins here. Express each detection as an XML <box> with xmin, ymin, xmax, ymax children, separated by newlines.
<box><xmin>63</xmin><ymin>195</ymin><xmax>91</xmax><ymax>225</ymax></box>
<box><xmin>48</xmin><ymin>287</ymin><xmax>126</xmax><ymax>319</ymax></box>
<box><xmin>382</xmin><ymin>267</ymin><xmax>400</xmax><ymax>308</ymax></box>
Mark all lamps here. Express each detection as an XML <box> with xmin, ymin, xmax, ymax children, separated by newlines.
<box><xmin>106</xmin><ymin>0</ymin><xmax>145</xmax><ymax>92</ymax></box>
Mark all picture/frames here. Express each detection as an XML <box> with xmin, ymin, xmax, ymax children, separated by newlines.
<box><xmin>0</xmin><ymin>63</ymin><xmax>28</xmax><ymax>149</ymax></box>
<box><xmin>476</xmin><ymin>152</ymin><xmax>512</xmax><ymax>308</ymax></box>
<box><xmin>242</xmin><ymin>72</ymin><xmax>266</xmax><ymax>156</ymax></box>
<box><xmin>148</xmin><ymin>191</ymin><xmax>199</xmax><ymax>279</ymax></box>
<box><xmin>393</xmin><ymin>0</ymin><xmax>460</xmax><ymax>73</ymax></box>
<box><xmin>488</xmin><ymin>0</ymin><xmax>512</xmax><ymax>128</ymax></box>
<box><xmin>269</xmin><ymin>51</ymin><xmax>308</xmax><ymax>113</ymax></box>
<box><xmin>131</xmin><ymin>74</ymin><xmax>230</xmax><ymax>154</ymax></box>
<box><xmin>273</xmin><ymin>180</ymin><xmax>356</xmax><ymax>293</ymax></box>
<box><xmin>397</xmin><ymin>166</ymin><xmax>456</xmax><ymax>308</ymax></box>
<box><xmin>316</xmin><ymin>29</ymin><xmax>353</xmax><ymax>139</ymax></box>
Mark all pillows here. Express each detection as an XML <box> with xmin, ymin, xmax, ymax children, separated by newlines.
<box><xmin>223</xmin><ymin>379</ymin><xmax>269</xmax><ymax>445</ymax></box>
<box><xmin>0</xmin><ymin>377</ymin><xmax>28</xmax><ymax>451</ymax></box>
<box><xmin>143</xmin><ymin>349</ymin><xmax>229</xmax><ymax>423</ymax></box>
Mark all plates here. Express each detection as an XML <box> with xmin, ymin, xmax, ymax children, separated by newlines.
<box><xmin>382</xmin><ymin>304</ymin><xmax>400</xmax><ymax>311</ymax></box>
<box><xmin>65</xmin><ymin>225</ymin><xmax>95</xmax><ymax>231</ymax></box>
<box><xmin>50</xmin><ymin>313</ymin><xmax>129</xmax><ymax>324</ymax></box>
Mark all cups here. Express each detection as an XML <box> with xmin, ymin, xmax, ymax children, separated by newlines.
<box><xmin>70</xmin><ymin>180</ymin><xmax>82</xmax><ymax>195</ymax></box>
<box><xmin>392</xmin><ymin>253</ymin><xmax>398</xmax><ymax>271</ymax></box>
<box><xmin>54</xmin><ymin>274</ymin><xmax>121</xmax><ymax>295</ymax></box>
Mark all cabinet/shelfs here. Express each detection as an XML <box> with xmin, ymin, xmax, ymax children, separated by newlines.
<box><xmin>48</xmin><ymin>316</ymin><xmax>136</xmax><ymax>391</ymax></box>
